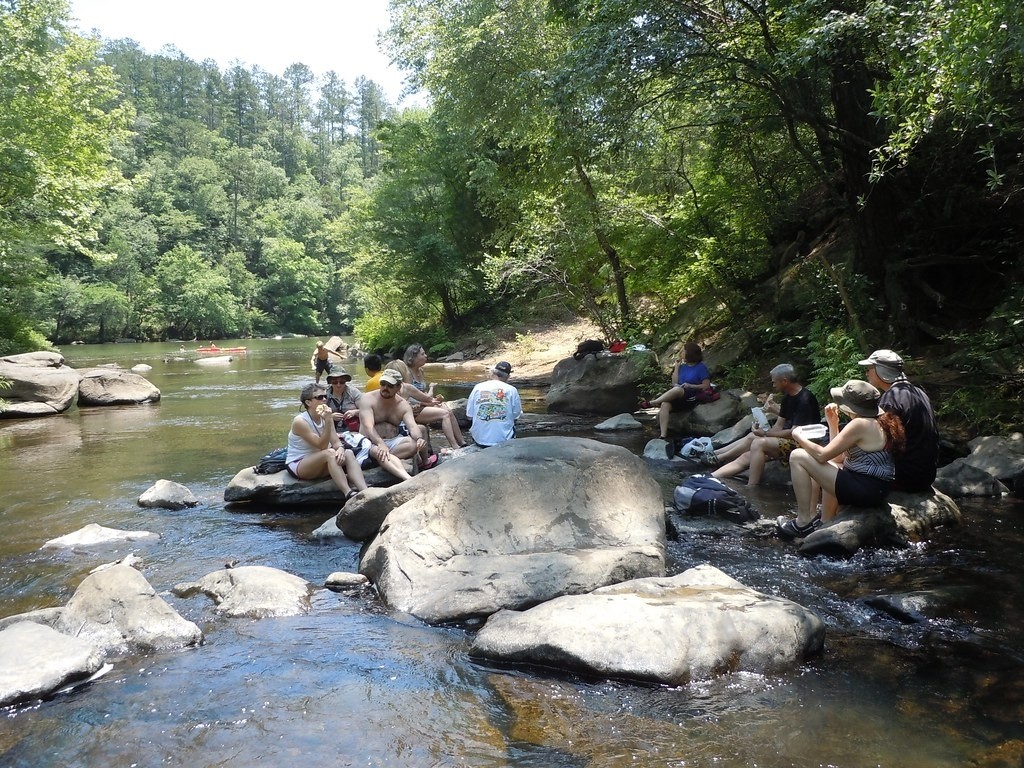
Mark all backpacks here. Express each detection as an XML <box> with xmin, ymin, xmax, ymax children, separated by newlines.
<box><xmin>674</xmin><ymin>471</ymin><xmax>752</xmax><ymax>523</ymax></box>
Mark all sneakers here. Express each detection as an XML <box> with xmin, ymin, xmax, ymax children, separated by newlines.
<box><xmin>638</xmin><ymin>400</ymin><xmax>655</xmax><ymax>409</ymax></box>
<box><xmin>810</xmin><ymin>514</ymin><xmax>823</xmax><ymax>529</ymax></box>
<box><xmin>418</xmin><ymin>452</ymin><xmax>439</xmax><ymax>470</ymax></box>
<box><xmin>655</xmin><ymin>436</ymin><xmax>668</xmax><ymax>439</ymax></box>
<box><xmin>358</xmin><ymin>453</ymin><xmax>378</xmax><ymax>471</ymax></box>
<box><xmin>777</xmin><ymin>514</ymin><xmax>816</xmax><ymax>539</ymax></box>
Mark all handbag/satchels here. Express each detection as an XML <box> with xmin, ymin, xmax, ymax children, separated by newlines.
<box><xmin>676</xmin><ymin>437</ymin><xmax>713</xmax><ymax>458</ymax></box>
<box><xmin>343</xmin><ymin>415</ymin><xmax>360</xmax><ymax>432</ymax></box>
<box><xmin>694</xmin><ymin>383</ymin><xmax>721</xmax><ymax>403</ymax></box>
<box><xmin>252</xmin><ymin>446</ymin><xmax>289</xmax><ymax>475</ymax></box>
<box><xmin>607</xmin><ymin>341</ymin><xmax>629</xmax><ymax>353</ymax></box>
<box><xmin>573</xmin><ymin>338</ymin><xmax>604</xmax><ymax>360</ymax></box>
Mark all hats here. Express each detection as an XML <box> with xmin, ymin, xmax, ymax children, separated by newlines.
<box><xmin>379</xmin><ymin>368</ymin><xmax>403</xmax><ymax>385</ymax></box>
<box><xmin>858</xmin><ymin>349</ymin><xmax>907</xmax><ymax>384</ymax></box>
<box><xmin>326</xmin><ymin>365</ymin><xmax>352</xmax><ymax>384</ymax></box>
<box><xmin>494</xmin><ymin>361</ymin><xmax>512</xmax><ymax>374</ymax></box>
<box><xmin>830</xmin><ymin>379</ymin><xmax>886</xmax><ymax>417</ymax></box>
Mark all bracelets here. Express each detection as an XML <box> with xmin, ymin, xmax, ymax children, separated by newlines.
<box><xmin>416</xmin><ymin>437</ymin><xmax>423</xmax><ymax>440</ymax></box>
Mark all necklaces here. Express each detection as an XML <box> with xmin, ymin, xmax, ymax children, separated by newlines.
<box><xmin>307</xmin><ymin>411</ymin><xmax>321</xmax><ymax>438</ymax></box>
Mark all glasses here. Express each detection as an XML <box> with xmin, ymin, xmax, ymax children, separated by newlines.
<box><xmin>330</xmin><ymin>380</ymin><xmax>347</xmax><ymax>384</ymax></box>
<box><xmin>307</xmin><ymin>394</ymin><xmax>328</xmax><ymax>401</ymax></box>
<box><xmin>380</xmin><ymin>381</ymin><xmax>398</xmax><ymax>388</ymax></box>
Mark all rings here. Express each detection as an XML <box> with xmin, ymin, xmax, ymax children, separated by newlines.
<box><xmin>350</xmin><ymin>416</ymin><xmax>352</xmax><ymax>418</ymax></box>
<box><xmin>348</xmin><ymin>415</ymin><xmax>350</xmax><ymax>416</ymax></box>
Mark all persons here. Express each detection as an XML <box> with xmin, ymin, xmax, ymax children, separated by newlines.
<box><xmin>180</xmin><ymin>345</ymin><xmax>185</xmax><ymax>351</ymax></box>
<box><xmin>403</xmin><ymin>344</ymin><xmax>470</xmax><ymax>449</ymax></box>
<box><xmin>692</xmin><ymin>363</ymin><xmax>852</xmax><ymax>485</ymax></box>
<box><xmin>359</xmin><ymin>369</ymin><xmax>427</xmax><ymax>480</ymax></box>
<box><xmin>364</xmin><ymin>354</ymin><xmax>384</xmax><ymax>393</ymax></box>
<box><xmin>383</xmin><ymin>359</ymin><xmax>438</xmax><ymax>469</ymax></box>
<box><xmin>210</xmin><ymin>341</ymin><xmax>216</xmax><ymax>347</ymax></box>
<box><xmin>820</xmin><ymin>349</ymin><xmax>939</xmax><ymax>523</ymax></box>
<box><xmin>324</xmin><ymin>365</ymin><xmax>363</xmax><ymax>431</ymax></box>
<box><xmin>466</xmin><ymin>361</ymin><xmax>523</xmax><ymax>448</ymax></box>
<box><xmin>311</xmin><ymin>341</ymin><xmax>344</xmax><ymax>383</ymax></box>
<box><xmin>781</xmin><ymin>379</ymin><xmax>896</xmax><ymax>539</ymax></box>
<box><xmin>285</xmin><ymin>383</ymin><xmax>367</xmax><ymax>500</ymax></box>
<box><xmin>640</xmin><ymin>343</ymin><xmax>711</xmax><ymax>439</ymax></box>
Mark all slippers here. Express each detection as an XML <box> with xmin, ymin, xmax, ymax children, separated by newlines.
<box><xmin>343</xmin><ymin>488</ymin><xmax>360</xmax><ymax>502</ymax></box>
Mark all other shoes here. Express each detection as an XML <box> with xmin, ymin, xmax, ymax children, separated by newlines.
<box><xmin>460</xmin><ymin>442</ymin><xmax>471</xmax><ymax>448</ymax></box>
<box><xmin>696</xmin><ymin>449</ymin><xmax>722</xmax><ymax>466</ymax></box>
